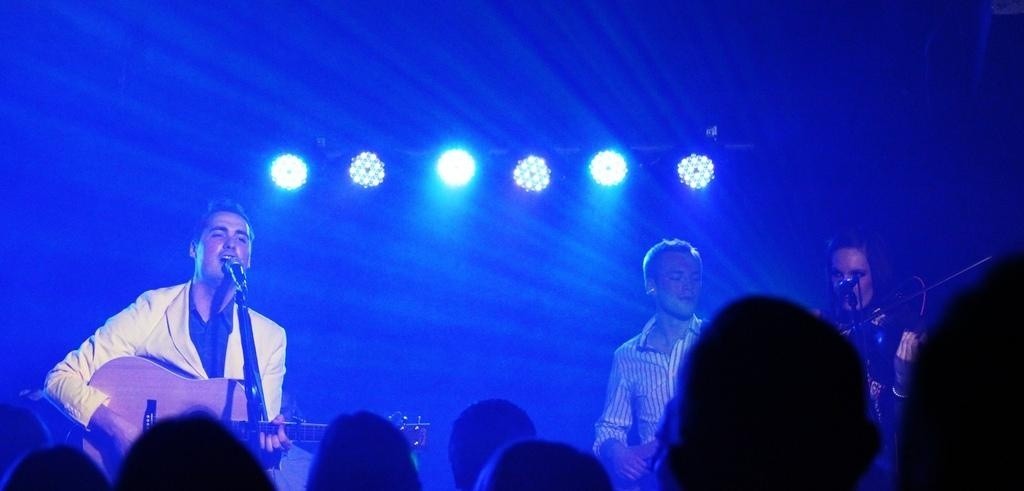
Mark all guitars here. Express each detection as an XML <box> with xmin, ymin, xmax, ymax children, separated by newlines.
<box><xmin>82</xmin><ymin>355</ymin><xmax>430</xmax><ymax>487</ymax></box>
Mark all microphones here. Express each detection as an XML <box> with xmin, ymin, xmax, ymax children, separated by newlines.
<box><xmin>835</xmin><ymin>274</ymin><xmax>862</xmax><ymax>295</ymax></box>
<box><xmin>222</xmin><ymin>256</ymin><xmax>249</xmax><ymax>295</ymax></box>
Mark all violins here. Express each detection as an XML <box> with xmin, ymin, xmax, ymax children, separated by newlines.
<box><xmin>843</xmin><ymin>304</ymin><xmax>932</xmax><ymax>350</ymax></box>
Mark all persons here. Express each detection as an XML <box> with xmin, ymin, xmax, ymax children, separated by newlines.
<box><xmin>805</xmin><ymin>227</ymin><xmax>928</xmax><ymax>486</ymax></box>
<box><xmin>41</xmin><ymin>198</ymin><xmax>293</xmax><ymax>490</ymax></box>
<box><xmin>0</xmin><ymin>247</ymin><xmax>1023</xmax><ymax>490</ymax></box>
<box><xmin>587</xmin><ymin>234</ymin><xmax>722</xmax><ymax>488</ymax></box>
